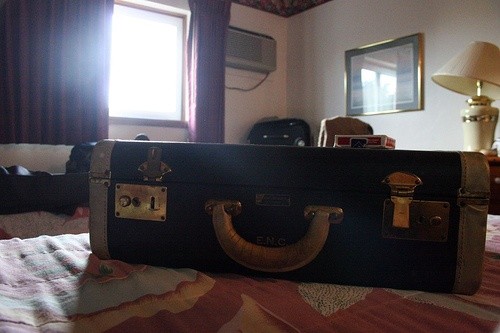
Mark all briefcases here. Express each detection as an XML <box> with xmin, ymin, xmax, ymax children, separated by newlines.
<box><xmin>87</xmin><ymin>139</ymin><xmax>491</xmax><ymax>295</ymax></box>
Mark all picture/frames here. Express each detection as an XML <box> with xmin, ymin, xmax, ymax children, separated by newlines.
<box><xmin>344</xmin><ymin>34</ymin><xmax>424</xmax><ymax>116</ymax></box>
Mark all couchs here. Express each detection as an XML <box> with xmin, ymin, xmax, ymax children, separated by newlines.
<box><xmin>2</xmin><ymin>141</ymin><xmax>95</xmax><ymax>241</ymax></box>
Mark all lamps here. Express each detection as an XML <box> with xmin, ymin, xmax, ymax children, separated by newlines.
<box><xmin>431</xmin><ymin>41</ymin><xmax>500</xmax><ymax>151</ymax></box>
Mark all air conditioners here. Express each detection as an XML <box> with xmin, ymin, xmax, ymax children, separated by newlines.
<box><xmin>223</xmin><ymin>25</ymin><xmax>277</xmax><ymax>75</ymax></box>
